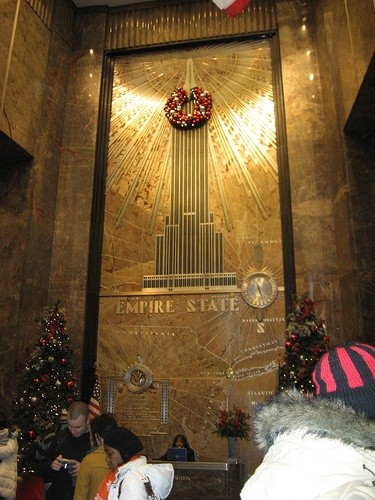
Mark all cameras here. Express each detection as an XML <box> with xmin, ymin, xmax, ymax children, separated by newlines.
<box><xmin>60</xmin><ymin>461</ymin><xmax>75</xmax><ymax>474</ymax></box>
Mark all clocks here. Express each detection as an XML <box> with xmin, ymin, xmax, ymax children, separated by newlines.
<box><xmin>242</xmin><ymin>272</ymin><xmax>277</xmax><ymax>307</ymax></box>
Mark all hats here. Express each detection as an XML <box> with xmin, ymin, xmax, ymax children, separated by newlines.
<box><xmin>104</xmin><ymin>426</ymin><xmax>144</xmax><ymax>455</ymax></box>
<box><xmin>312</xmin><ymin>342</ymin><xmax>375</xmax><ymax>419</ymax></box>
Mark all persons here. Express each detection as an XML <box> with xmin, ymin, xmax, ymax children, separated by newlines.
<box><xmin>239</xmin><ymin>342</ymin><xmax>375</xmax><ymax>500</ymax></box>
<box><xmin>42</xmin><ymin>402</ymin><xmax>98</xmax><ymax>500</ymax></box>
<box><xmin>73</xmin><ymin>413</ymin><xmax>118</xmax><ymax>500</ymax></box>
<box><xmin>149</xmin><ymin>434</ymin><xmax>195</xmax><ymax>462</ymax></box>
<box><xmin>0</xmin><ymin>411</ymin><xmax>18</xmax><ymax>500</ymax></box>
<box><xmin>95</xmin><ymin>426</ymin><xmax>174</xmax><ymax>500</ymax></box>
